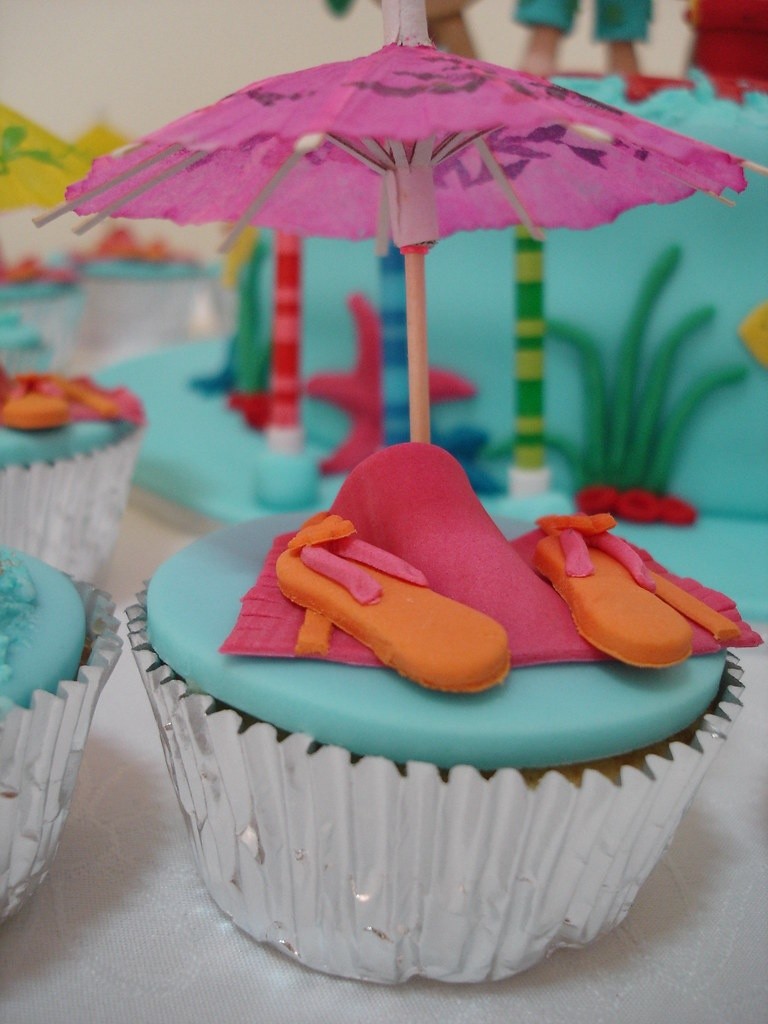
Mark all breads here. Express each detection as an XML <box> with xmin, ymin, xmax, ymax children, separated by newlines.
<box><xmin>0</xmin><ymin>222</ymin><xmax>767</xmax><ymax>982</ymax></box>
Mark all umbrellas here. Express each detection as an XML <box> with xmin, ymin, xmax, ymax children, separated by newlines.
<box><xmin>32</xmin><ymin>0</ymin><xmax>768</xmax><ymax>444</ymax></box>
